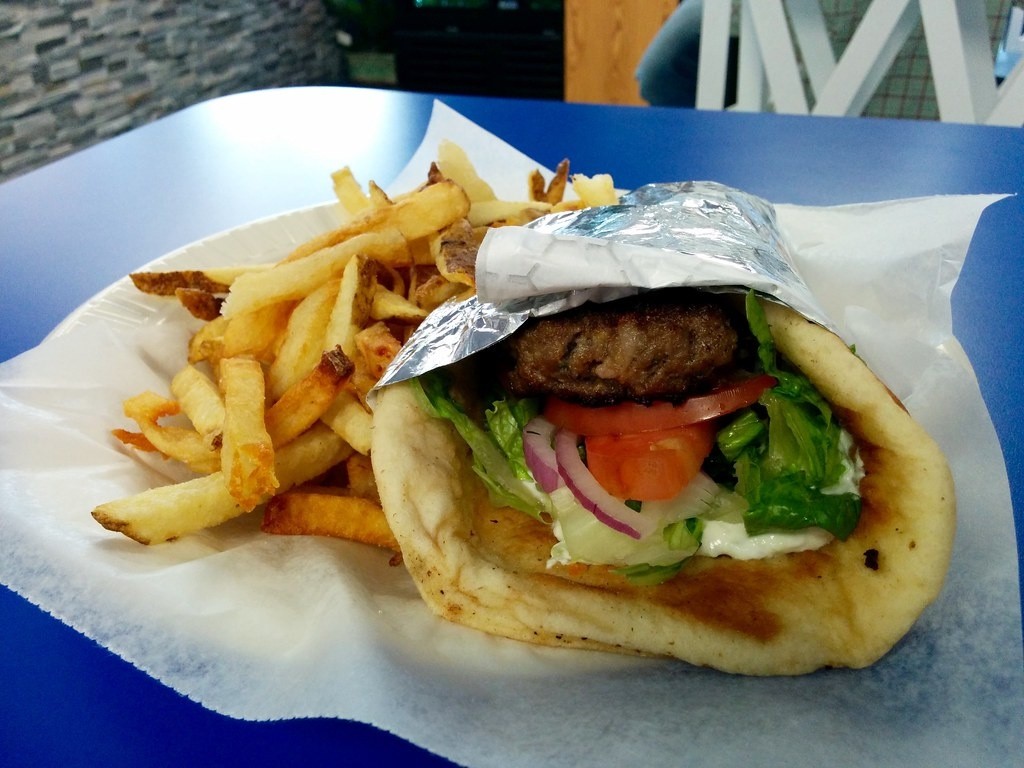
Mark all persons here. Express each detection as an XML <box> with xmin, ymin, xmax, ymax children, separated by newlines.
<box><xmin>634</xmin><ymin>0</ymin><xmax>739</xmax><ymax>111</ymax></box>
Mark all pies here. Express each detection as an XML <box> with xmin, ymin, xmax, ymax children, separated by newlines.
<box><xmin>368</xmin><ymin>290</ymin><xmax>958</xmax><ymax>680</ymax></box>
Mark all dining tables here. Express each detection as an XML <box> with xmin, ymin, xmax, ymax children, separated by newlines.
<box><xmin>0</xmin><ymin>87</ymin><xmax>1022</xmax><ymax>766</ymax></box>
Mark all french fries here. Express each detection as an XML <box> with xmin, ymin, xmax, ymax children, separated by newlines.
<box><xmin>87</xmin><ymin>130</ymin><xmax>621</xmax><ymax>552</ymax></box>
